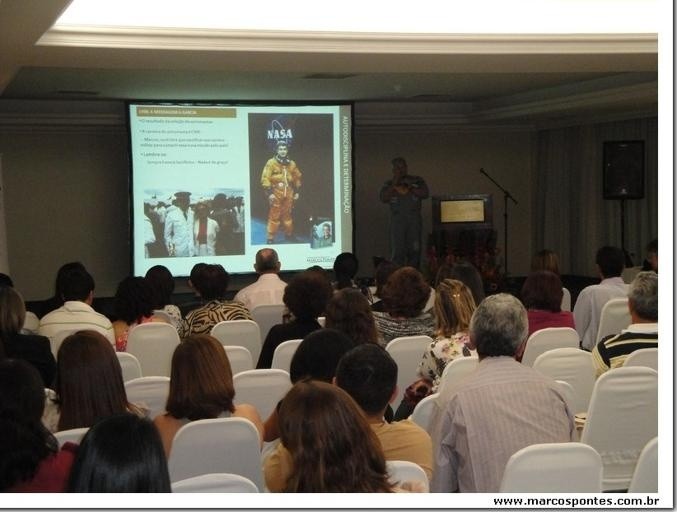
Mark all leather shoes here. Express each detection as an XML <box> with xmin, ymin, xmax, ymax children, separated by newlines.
<box><xmin>267</xmin><ymin>239</ymin><xmax>274</xmax><ymax>244</ymax></box>
<box><xmin>284</xmin><ymin>233</ymin><xmax>301</xmax><ymax>243</ymax></box>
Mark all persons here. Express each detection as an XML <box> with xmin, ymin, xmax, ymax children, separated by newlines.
<box><xmin>320</xmin><ymin>224</ymin><xmax>332</xmax><ymax>248</ymax></box>
<box><xmin>378</xmin><ymin>159</ymin><xmax>428</xmax><ymax>269</ymax></box>
<box><xmin>143</xmin><ymin>191</ymin><xmax>249</xmax><ymax>255</ymax></box>
<box><xmin>261</xmin><ymin>141</ymin><xmax>303</xmax><ymax>244</ymax></box>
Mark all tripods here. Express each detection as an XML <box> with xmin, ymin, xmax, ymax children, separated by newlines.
<box><xmin>620</xmin><ymin>199</ymin><xmax>633</xmax><ymax>268</ymax></box>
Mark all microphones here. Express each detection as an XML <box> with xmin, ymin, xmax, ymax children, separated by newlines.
<box><xmin>479</xmin><ymin>167</ymin><xmax>488</xmax><ymax>177</ymax></box>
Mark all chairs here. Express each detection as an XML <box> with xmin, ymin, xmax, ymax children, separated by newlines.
<box><xmin>0</xmin><ymin>265</ymin><xmax>659</xmax><ymax>493</ymax></box>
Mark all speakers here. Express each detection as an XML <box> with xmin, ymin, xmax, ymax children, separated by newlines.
<box><xmin>602</xmin><ymin>140</ymin><xmax>646</xmax><ymax>200</ymax></box>
<box><xmin>432</xmin><ymin>193</ymin><xmax>493</xmax><ymax>231</ymax></box>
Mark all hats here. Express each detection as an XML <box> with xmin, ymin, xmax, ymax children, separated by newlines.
<box><xmin>195</xmin><ymin>202</ymin><xmax>207</xmax><ymax>210</ymax></box>
<box><xmin>174</xmin><ymin>191</ymin><xmax>192</xmax><ymax>198</ymax></box>
